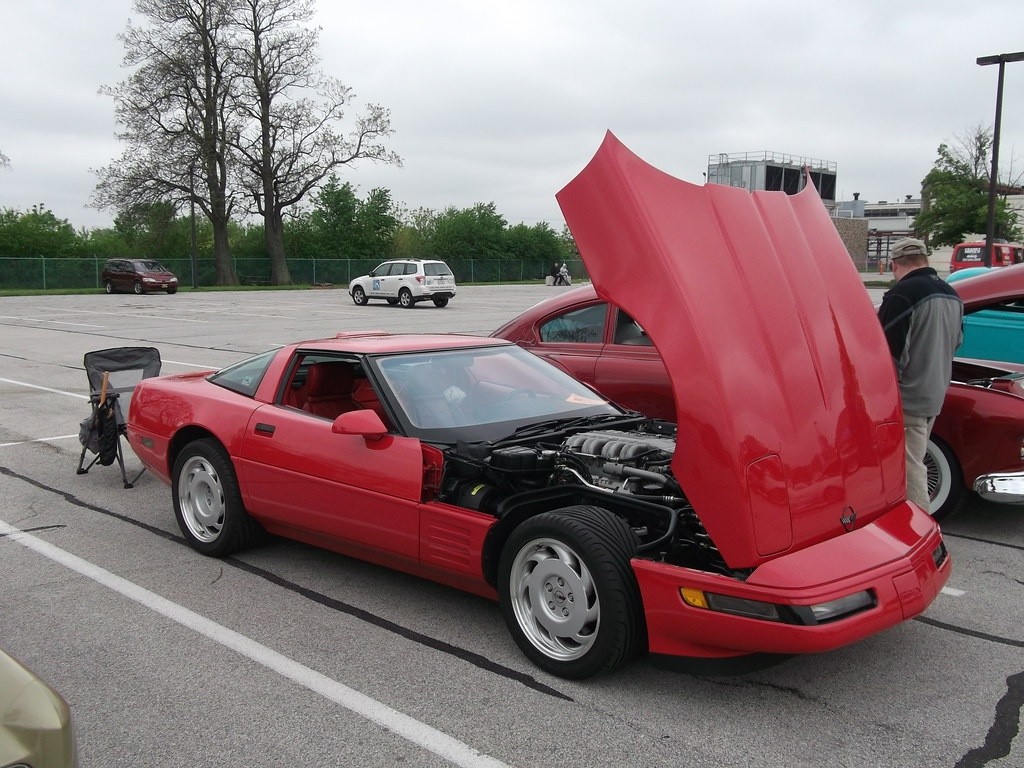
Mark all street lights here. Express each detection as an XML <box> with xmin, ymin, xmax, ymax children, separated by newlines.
<box><xmin>977</xmin><ymin>52</ymin><xmax>1024</xmax><ymax>268</ymax></box>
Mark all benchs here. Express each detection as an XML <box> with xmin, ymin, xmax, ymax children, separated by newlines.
<box><xmin>546</xmin><ymin>275</ymin><xmax>571</xmax><ymax>286</ymax></box>
<box><xmin>574</xmin><ymin>323</ymin><xmax>651</xmax><ymax>344</ymax></box>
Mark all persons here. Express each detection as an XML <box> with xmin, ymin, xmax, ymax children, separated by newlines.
<box><xmin>560</xmin><ymin>264</ymin><xmax>571</xmax><ymax>286</ymax></box>
<box><xmin>877</xmin><ymin>237</ymin><xmax>964</xmax><ymax>512</ymax></box>
<box><xmin>551</xmin><ymin>263</ymin><xmax>562</xmax><ymax>285</ymax></box>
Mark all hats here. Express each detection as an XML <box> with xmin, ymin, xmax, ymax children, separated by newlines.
<box><xmin>890</xmin><ymin>237</ymin><xmax>928</xmax><ymax>261</ymax></box>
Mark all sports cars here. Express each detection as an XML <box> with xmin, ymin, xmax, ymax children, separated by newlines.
<box><xmin>123</xmin><ymin>128</ymin><xmax>950</xmax><ymax>682</ymax></box>
<box><xmin>465</xmin><ymin>254</ymin><xmax>1024</xmax><ymax>513</ymax></box>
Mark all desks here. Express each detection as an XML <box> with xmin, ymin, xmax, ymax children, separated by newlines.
<box><xmin>241</xmin><ymin>276</ymin><xmax>270</xmax><ymax>286</ymax></box>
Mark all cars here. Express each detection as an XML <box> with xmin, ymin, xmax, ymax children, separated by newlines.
<box><xmin>102</xmin><ymin>258</ymin><xmax>178</xmax><ymax>295</ymax></box>
<box><xmin>941</xmin><ymin>265</ymin><xmax>1024</xmax><ymax>363</ymax></box>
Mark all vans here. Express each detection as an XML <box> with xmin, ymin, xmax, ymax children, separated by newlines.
<box><xmin>949</xmin><ymin>242</ymin><xmax>1024</xmax><ymax>278</ymax></box>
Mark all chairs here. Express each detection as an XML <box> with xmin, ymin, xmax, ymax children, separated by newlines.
<box><xmin>76</xmin><ymin>347</ymin><xmax>162</xmax><ymax>490</ymax></box>
<box><xmin>290</xmin><ymin>360</ymin><xmax>397</xmax><ymax>432</ymax></box>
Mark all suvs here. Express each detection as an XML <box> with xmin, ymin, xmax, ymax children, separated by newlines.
<box><xmin>347</xmin><ymin>258</ymin><xmax>457</xmax><ymax>309</ymax></box>
